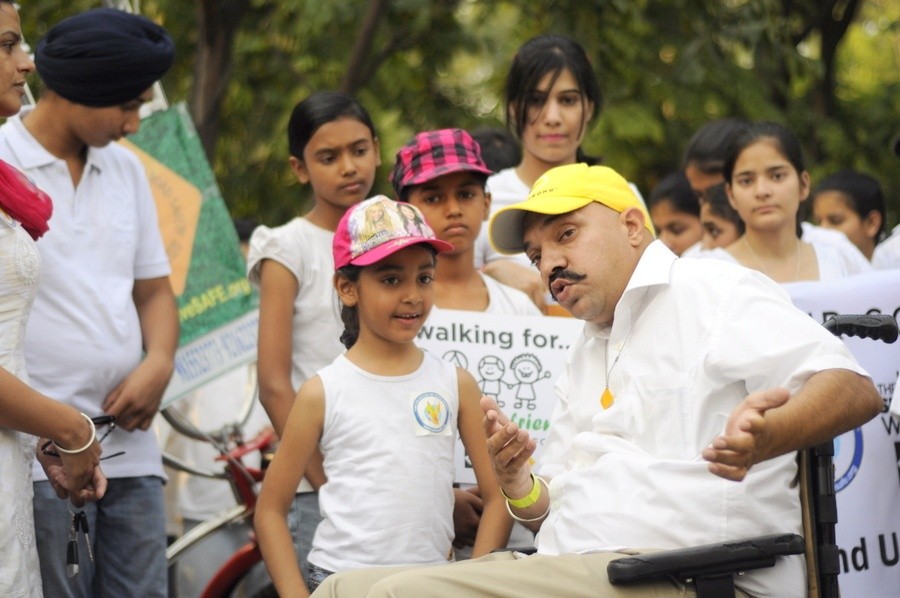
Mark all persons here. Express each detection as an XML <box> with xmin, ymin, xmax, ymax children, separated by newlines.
<box><xmin>0</xmin><ymin>14</ymin><xmax>900</xmax><ymax>598</ymax></box>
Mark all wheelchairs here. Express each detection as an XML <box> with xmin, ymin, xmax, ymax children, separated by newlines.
<box><xmin>606</xmin><ymin>314</ymin><xmax>900</xmax><ymax>598</ymax></box>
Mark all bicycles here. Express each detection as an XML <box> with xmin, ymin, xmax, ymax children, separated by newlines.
<box><xmin>158</xmin><ymin>367</ymin><xmax>276</xmax><ymax>598</ymax></box>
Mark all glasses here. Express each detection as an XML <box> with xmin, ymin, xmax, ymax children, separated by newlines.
<box><xmin>40</xmin><ymin>414</ymin><xmax>126</xmax><ymax>461</ymax></box>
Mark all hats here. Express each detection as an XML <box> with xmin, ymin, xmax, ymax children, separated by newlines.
<box><xmin>387</xmin><ymin>127</ymin><xmax>493</xmax><ymax>196</ymax></box>
<box><xmin>34</xmin><ymin>7</ymin><xmax>176</xmax><ymax>109</ymax></box>
<box><xmin>332</xmin><ymin>193</ymin><xmax>454</xmax><ymax>269</ymax></box>
<box><xmin>489</xmin><ymin>160</ymin><xmax>658</xmax><ymax>254</ymax></box>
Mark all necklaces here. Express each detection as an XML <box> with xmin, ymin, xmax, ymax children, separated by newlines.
<box><xmin>600</xmin><ymin>330</ymin><xmax>632</xmax><ymax>410</ymax></box>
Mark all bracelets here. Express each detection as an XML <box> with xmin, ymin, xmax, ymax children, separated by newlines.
<box><xmin>500</xmin><ymin>455</ymin><xmax>540</xmax><ymax>509</ymax></box>
<box><xmin>453</xmin><ymin>481</ymin><xmax>460</xmax><ymax>489</ymax></box>
<box><xmin>51</xmin><ymin>410</ymin><xmax>97</xmax><ymax>452</ymax></box>
<box><xmin>504</xmin><ymin>475</ymin><xmax>549</xmax><ymax>522</ymax></box>
